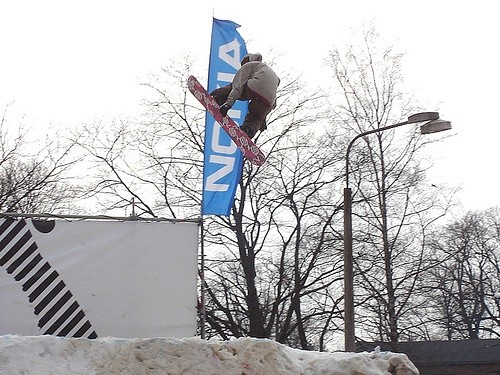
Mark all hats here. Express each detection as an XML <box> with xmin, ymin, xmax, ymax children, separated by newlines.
<box><xmin>240</xmin><ymin>54</ymin><xmax>250</xmax><ymax>67</ymax></box>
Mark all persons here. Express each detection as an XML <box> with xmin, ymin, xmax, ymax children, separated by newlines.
<box><xmin>210</xmin><ymin>53</ymin><xmax>281</xmax><ymax>138</ymax></box>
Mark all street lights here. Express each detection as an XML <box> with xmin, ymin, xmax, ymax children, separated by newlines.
<box><xmin>344</xmin><ymin>112</ymin><xmax>451</xmax><ymax>352</ymax></box>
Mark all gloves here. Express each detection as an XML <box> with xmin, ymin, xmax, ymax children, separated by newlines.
<box><xmin>258</xmin><ymin>119</ymin><xmax>266</xmax><ymax>131</ymax></box>
<box><xmin>220</xmin><ymin>104</ymin><xmax>230</xmax><ymax>117</ymax></box>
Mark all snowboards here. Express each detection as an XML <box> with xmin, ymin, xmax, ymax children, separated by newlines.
<box><xmin>186</xmin><ymin>75</ymin><xmax>266</xmax><ymax>166</ymax></box>
